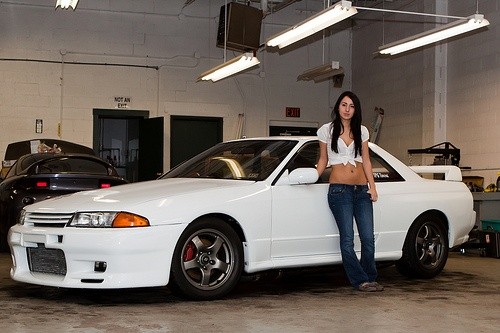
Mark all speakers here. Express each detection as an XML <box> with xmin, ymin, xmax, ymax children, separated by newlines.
<box><xmin>216</xmin><ymin>1</ymin><xmax>264</xmax><ymax>55</ymax></box>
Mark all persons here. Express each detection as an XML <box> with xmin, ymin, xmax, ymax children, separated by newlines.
<box><xmin>316</xmin><ymin>91</ymin><xmax>384</xmax><ymax>293</ymax></box>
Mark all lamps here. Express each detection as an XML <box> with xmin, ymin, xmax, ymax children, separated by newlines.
<box><xmin>296</xmin><ymin>0</ymin><xmax>344</xmax><ymax>82</ymax></box>
<box><xmin>198</xmin><ymin>0</ymin><xmax>261</xmax><ymax>82</ymax></box>
<box><xmin>56</xmin><ymin>0</ymin><xmax>79</xmax><ymax>10</ymax></box>
<box><xmin>370</xmin><ymin>0</ymin><xmax>490</xmax><ymax>56</ymax></box>
<box><xmin>265</xmin><ymin>0</ymin><xmax>358</xmax><ymax>50</ymax></box>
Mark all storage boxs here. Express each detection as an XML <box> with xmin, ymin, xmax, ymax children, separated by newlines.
<box><xmin>477</xmin><ymin>219</ymin><xmax>500</xmax><ymax>258</ymax></box>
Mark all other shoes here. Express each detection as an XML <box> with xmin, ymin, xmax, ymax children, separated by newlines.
<box><xmin>358</xmin><ymin>282</ymin><xmax>376</xmax><ymax>291</ymax></box>
<box><xmin>371</xmin><ymin>282</ymin><xmax>384</xmax><ymax>291</ymax></box>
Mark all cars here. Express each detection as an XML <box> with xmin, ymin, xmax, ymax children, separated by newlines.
<box><xmin>0</xmin><ymin>139</ymin><xmax>128</xmax><ymax>247</ymax></box>
<box><xmin>7</xmin><ymin>135</ymin><xmax>476</xmax><ymax>300</ymax></box>
